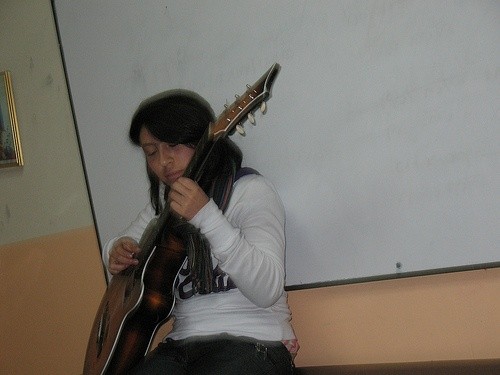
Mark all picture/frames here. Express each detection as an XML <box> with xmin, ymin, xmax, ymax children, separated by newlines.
<box><xmin>0</xmin><ymin>71</ymin><xmax>25</xmax><ymax>169</ymax></box>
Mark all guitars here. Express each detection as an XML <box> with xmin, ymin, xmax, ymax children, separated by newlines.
<box><xmin>82</xmin><ymin>62</ymin><xmax>282</xmax><ymax>375</ymax></box>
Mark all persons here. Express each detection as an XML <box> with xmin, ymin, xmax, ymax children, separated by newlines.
<box><xmin>101</xmin><ymin>94</ymin><xmax>298</xmax><ymax>375</ymax></box>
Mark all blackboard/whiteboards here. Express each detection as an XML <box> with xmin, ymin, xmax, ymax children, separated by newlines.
<box><xmin>52</xmin><ymin>0</ymin><xmax>500</xmax><ymax>299</ymax></box>
